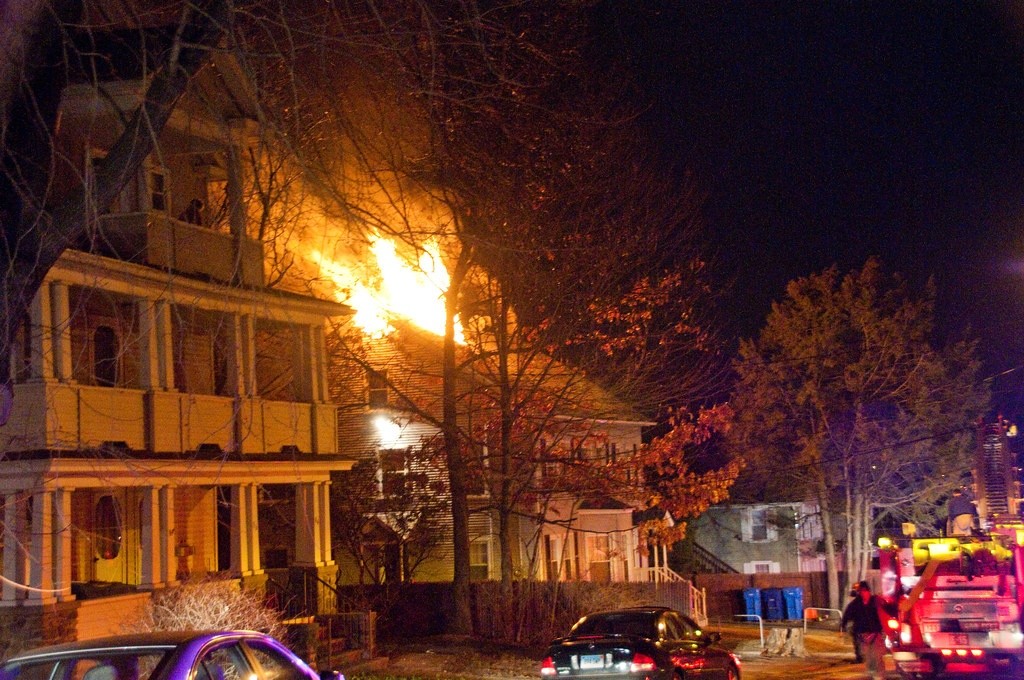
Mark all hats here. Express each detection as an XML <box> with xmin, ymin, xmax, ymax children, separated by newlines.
<box><xmin>857</xmin><ymin>581</ymin><xmax>871</xmax><ymax>590</ymax></box>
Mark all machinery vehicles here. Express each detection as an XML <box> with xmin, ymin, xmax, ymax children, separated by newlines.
<box><xmin>874</xmin><ymin>527</ymin><xmax>1024</xmax><ymax>680</ymax></box>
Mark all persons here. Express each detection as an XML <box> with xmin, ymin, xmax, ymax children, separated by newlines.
<box><xmin>840</xmin><ymin>580</ymin><xmax>898</xmax><ymax>673</ymax></box>
<box><xmin>179</xmin><ymin>199</ymin><xmax>204</xmax><ymax>226</ymax></box>
<box><xmin>948</xmin><ymin>486</ymin><xmax>980</xmax><ymax>529</ymax></box>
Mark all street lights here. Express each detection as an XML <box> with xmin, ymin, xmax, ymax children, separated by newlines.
<box><xmin>997</xmin><ymin>412</ymin><xmax>1018</xmax><ymax>515</ymax></box>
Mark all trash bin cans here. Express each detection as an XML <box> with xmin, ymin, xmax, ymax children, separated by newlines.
<box><xmin>783</xmin><ymin>586</ymin><xmax>803</xmax><ymax>620</ymax></box>
<box><xmin>744</xmin><ymin>588</ymin><xmax>762</xmax><ymax>621</ymax></box>
<box><xmin>762</xmin><ymin>588</ymin><xmax>785</xmax><ymax>621</ymax></box>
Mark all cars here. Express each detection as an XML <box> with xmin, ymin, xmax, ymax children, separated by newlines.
<box><xmin>0</xmin><ymin>630</ymin><xmax>345</xmax><ymax>680</ymax></box>
<box><xmin>541</xmin><ymin>605</ymin><xmax>742</xmax><ymax>680</ymax></box>
